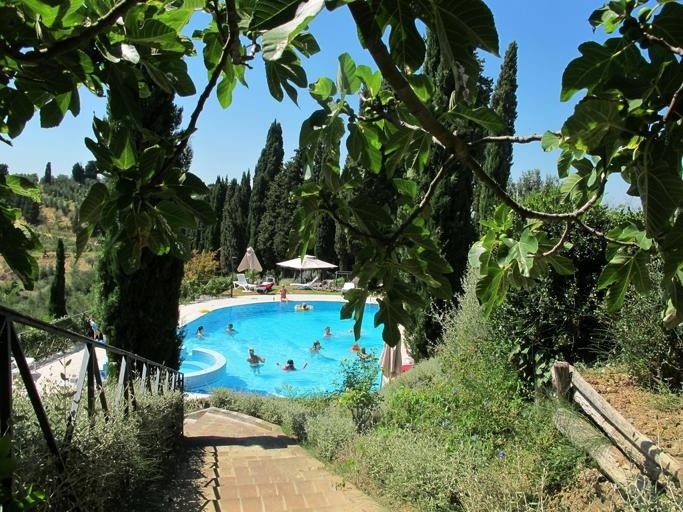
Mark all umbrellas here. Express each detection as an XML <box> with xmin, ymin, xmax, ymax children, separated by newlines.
<box><xmin>376</xmin><ymin>296</ymin><xmax>415</xmax><ymax>385</ymax></box>
<box><xmin>236</xmin><ymin>246</ymin><xmax>263</xmax><ymax>283</ymax></box>
<box><xmin>273</xmin><ymin>255</ymin><xmax>339</xmax><ymax>283</ymax></box>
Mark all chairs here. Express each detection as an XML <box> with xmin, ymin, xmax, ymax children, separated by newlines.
<box><xmin>10</xmin><ymin>354</ymin><xmax>38</xmax><ymax>383</ymax></box>
<box><xmin>232</xmin><ymin>271</ymin><xmax>355</xmax><ymax>294</ymax></box>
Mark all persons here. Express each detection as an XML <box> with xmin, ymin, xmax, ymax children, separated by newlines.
<box><xmin>89</xmin><ymin>316</ymin><xmax>101</xmax><ymax>341</ymax></box>
<box><xmin>246</xmin><ymin>347</ymin><xmax>265</xmax><ymax>375</ymax></box>
<box><xmin>348</xmin><ymin>342</ymin><xmax>359</xmax><ymax>353</ymax></box>
<box><xmin>272</xmin><ymin>359</ymin><xmax>308</xmax><ymax>370</ymax></box>
<box><xmin>223</xmin><ymin>324</ymin><xmax>239</xmax><ymax>338</ymax></box>
<box><xmin>196</xmin><ymin>325</ymin><xmax>207</xmax><ymax>339</ymax></box>
<box><xmin>308</xmin><ymin>340</ymin><xmax>334</xmax><ymax>360</ymax></box>
<box><xmin>358</xmin><ymin>347</ymin><xmax>370</xmax><ymax>359</ymax></box>
<box><xmin>295</xmin><ymin>302</ymin><xmax>313</xmax><ymax>311</ymax></box>
<box><xmin>278</xmin><ymin>284</ymin><xmax>291</xmax><ymax>306</ymax></box>
<box><xmin>322</xmin><ymin>326</ymin><xmax>337</xmax><ymax>338</ymax></box>
